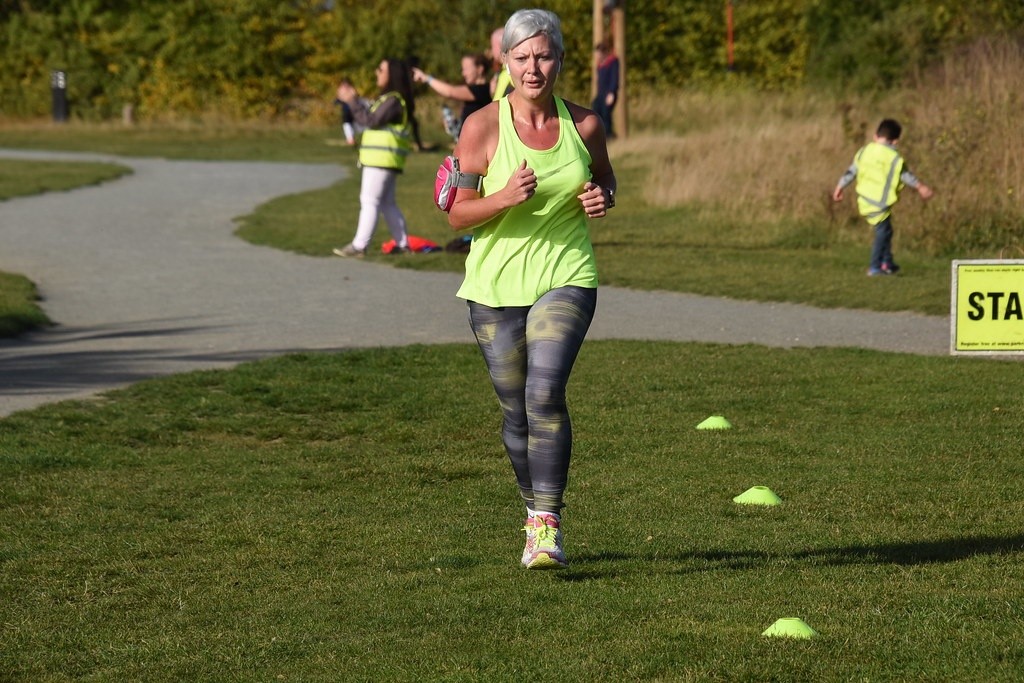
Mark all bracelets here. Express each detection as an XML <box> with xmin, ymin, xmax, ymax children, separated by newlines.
<box><xmin>426</xmin><ymin>75</ymin><xmax>432</xmax><ymax>84</ymax></box>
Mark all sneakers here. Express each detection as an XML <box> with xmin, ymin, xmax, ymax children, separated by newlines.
<box><xmin>526</xmin><ymin>514</ymin><xmax>565</xmax><ymax>570</ymax></box>
<box><xmin>521</xmin><ymin>518</ymin><xmax>535</xmax><ymax>565</ymax></box>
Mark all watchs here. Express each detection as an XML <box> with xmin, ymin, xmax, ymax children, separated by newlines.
<box><xmin>604</xmin><ymin>187</ymin><xmax>615</xmax><ymax>209</ymax></box>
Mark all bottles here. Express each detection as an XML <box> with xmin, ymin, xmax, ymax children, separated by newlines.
<box><xmin>442</xmin><ymin>104</ymin><xmax>459</xmax><ymax>137</ymax></box>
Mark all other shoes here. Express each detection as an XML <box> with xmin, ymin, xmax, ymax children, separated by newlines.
<box><xmin>390</xmin><ymin>247</ymin><xmax>412</xmax><ymax>255</ymax></box>
<box><xmin>869</xmin><ymin>268</ymin><xmax>887</xmax><ymax>276</ymax></box>
<box><xmin>886</xmin><ymin>265</ymin><xmax>900</xmax><ymax>273</ymax></box>
<box><xmin>333</xmin><ymin>243</ymin><xmax>365</xmax><ymax>258</ymax></box>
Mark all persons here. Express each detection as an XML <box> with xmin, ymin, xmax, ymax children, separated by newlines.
<box><xmin>435</xmin><ymin>9</ymin><xmax>617</xmax><ymax>569</ymax></box>
<box><xmin>324</xmin><ymin>27</ymin><xmax>619</xmax><ymax>260</ymax></box>
<box><xmin>833</xmin><ymin>118</ymin><xmax>933</xmax><ymax>276</ymax></box>
